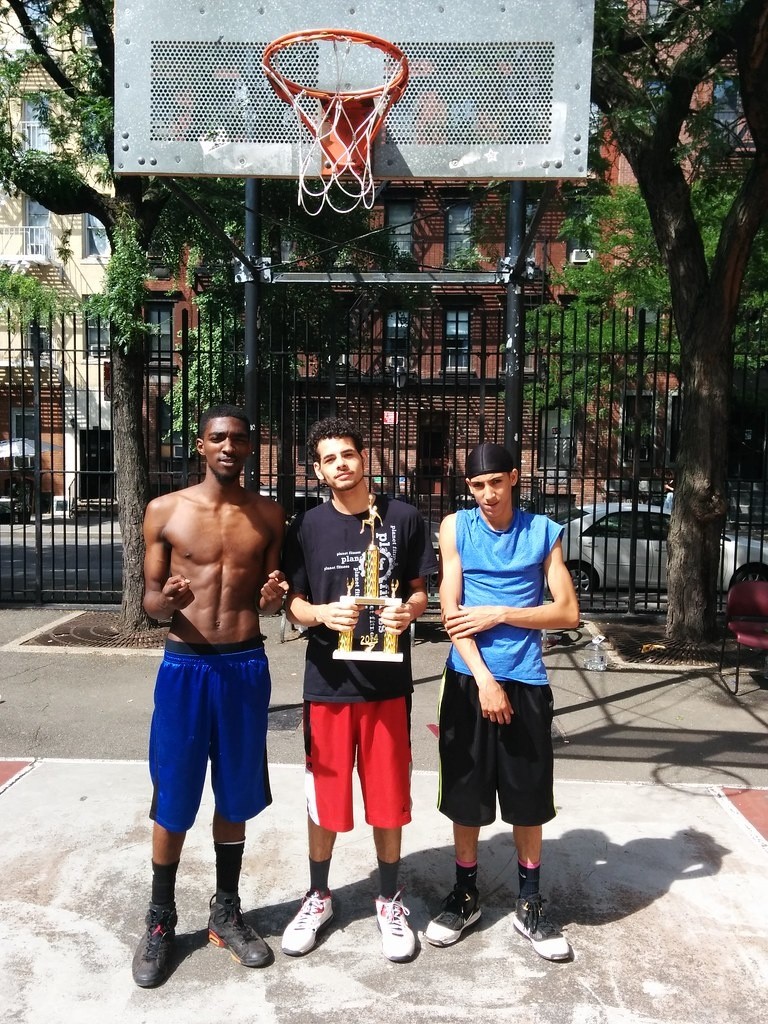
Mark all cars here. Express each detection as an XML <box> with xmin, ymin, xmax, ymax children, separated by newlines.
<box><xmin>251</xmin><ymin>485</ymin><xmax>444</xmax><ymax>588</ymax></box>
<box><xmin>545</xmin><ymin>502</ymin><xmax>768</xmax><ymax>595</ymax></box>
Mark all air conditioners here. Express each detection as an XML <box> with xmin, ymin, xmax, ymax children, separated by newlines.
<box><xmin>90</xmin><ymin>346</ymin><xmax>106</xmax><ymax>357</ymax></box>
<box><xmin>27</xmin><ymin>244</ymin><xmax>43</xmax><ymax>254</ymax></box>
<box><xmin>389</xmin><ymin>356</ymin><xmax>405</xmax><ymax>368</ymax></box>
<box><xmin>627</xmin><ymin>446</ymin><xmax>648</xmax><ymax>462</ymax></box>
<box><xmin>173</xmin><ymin>445</ymin><xmax>190</xmax><ymax>458</ymax></box>
<box><xmin>573</xmin><ymin>248</ymin><xmax>592</xmax><ymax>262</ymax></box>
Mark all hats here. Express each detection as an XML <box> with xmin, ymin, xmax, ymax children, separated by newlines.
<box><xmin>465</xmin><ymin>442</ymin><xmax>513</xmax><ymax>478</ymax></box>
<box><xmin>664</xmin><ymin>480</ymin><xmax>675</xmax><ymax>491</ymax></box>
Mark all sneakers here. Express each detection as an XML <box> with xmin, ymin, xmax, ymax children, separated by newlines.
<box><xmin>374</xmin><ymin>894</ymin><xmax>415</xmax><ymax>963</ymax></box>
<box><xmin>514</xmin><ymin>894</ymin><xmax>569</xmax><ymax>960</ymax></box>
<box><xmin>282</xmin><ymin>888</ymin><xmax>334</xmax><ymax>956</ymax></box>
<box><xmin>208</xmin><ymin>893</ymin><xmax>270</xmax><ymax>966</ymax></box>
<box><xmin>425</xmin><ymin>884</ymin><xmax>483</xmax><ymax>947</ymax></box>
<box><xmin>131</xmin><ymin>902</ymin><xmax>178</xmax><ymax>987</ymax></box>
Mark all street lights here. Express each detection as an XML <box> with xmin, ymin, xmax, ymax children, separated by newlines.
<box><xmin>389</xmin><ymin>367</ymin><xmax>408</xmax><ymax>499</ymax></box>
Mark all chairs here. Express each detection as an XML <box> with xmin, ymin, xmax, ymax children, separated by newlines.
<box><xmin>718</xmin><ymin>581</ymin><xmax>768</xmax><ymax>694</ymax></box>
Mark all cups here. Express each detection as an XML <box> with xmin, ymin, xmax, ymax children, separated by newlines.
<box><xmin>575</xmin><ymin>642</ymin><xmax>608</xmax><ymax>671</ymax></box>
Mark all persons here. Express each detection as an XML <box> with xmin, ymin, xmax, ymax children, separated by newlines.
<box><xmin>132</xmin><ymin>403</ymin><xmax>289</xmax><ymax>987</ymax></box>
<box><xmin>663</xmin><ymin>471</ymin><xmax>673</xmax><ymax>509</ymax></box>
<box><xmin>424</xmin><ymin>442</ymin><xmax>581</xmax><ymax>959</ymax></box>
<box><xmin>281</xmin><ymin>415</ymin><xmax>439</xmax><ymax>961</ymax></box>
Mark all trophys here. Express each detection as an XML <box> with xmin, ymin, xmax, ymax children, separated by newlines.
<box><xmin>332</xmin><ymin>493</ymin><xmax>404</xmax><ymax>662</ymax></box>
